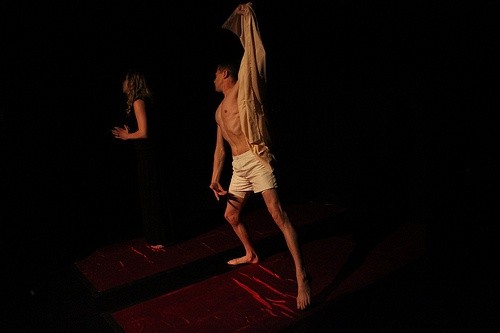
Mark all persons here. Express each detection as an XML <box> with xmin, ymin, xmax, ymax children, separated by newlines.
<box><xmin>209</xmin><ymin>0</ymin><xmax>313</xmax><ymax>311</ymax></box>
<box><xmin>107</xmin><ymin>68</ymin><xmax>169</xmax><ymax>250</ymax></box>
